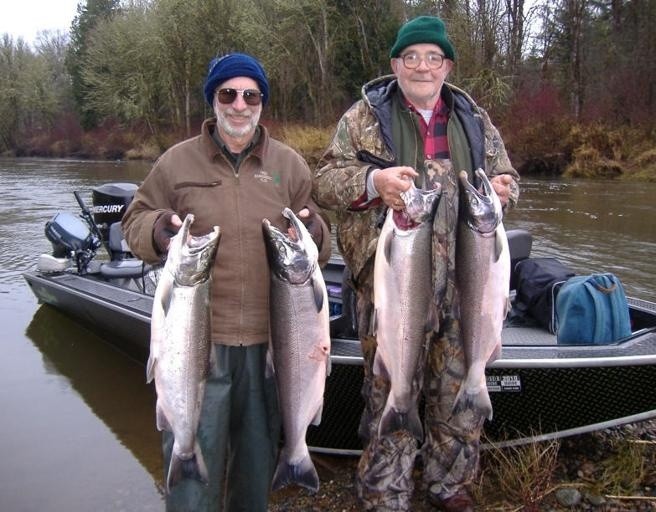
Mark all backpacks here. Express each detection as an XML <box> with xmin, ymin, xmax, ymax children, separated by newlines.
<box><xmin>507</xmin><ymin>258</ymin><xmax>632</xmax><ymax>344</ymax></box>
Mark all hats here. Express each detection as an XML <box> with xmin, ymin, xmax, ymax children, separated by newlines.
<box><xmin>205</xmin><ymin>52</ymin><xmax>269</xmax><ymax>105</ymax></box>
<box><xmin>389</xmin><ymin>16</ymin><xmax>453</xmax><ymax>59</ymax></box>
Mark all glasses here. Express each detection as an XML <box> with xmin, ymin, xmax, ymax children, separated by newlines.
<box><xmin>212</xmin><ymin>88</ymin><xmax>263</xmax><ymax>105</ymax></box>
<box><xmin>398</xmin><ymin>54</ymin><xmax>449</xmax><ymax>69</ymax></box>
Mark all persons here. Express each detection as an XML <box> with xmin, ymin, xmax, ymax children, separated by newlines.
<box><xmin>310</xmin><ymin>14</ymin><xmax>523</xmax><ymax>512</ymax></box>
<box><xmin>120</xmin><ymin>54</ymin><xmax>334</xmax><ymax>512</ymax></box>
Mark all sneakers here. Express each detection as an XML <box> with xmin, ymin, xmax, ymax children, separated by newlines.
<box><xmin>441</xmin><ymin>495</ymin><xmax>473</xmax><ymax>512</ymax></box>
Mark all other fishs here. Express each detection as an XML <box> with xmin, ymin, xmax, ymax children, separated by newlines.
<box><xmin>257</xmin><ymin>206</ymin><xmax>334</xmax><ymax>493</ymax></box>
<box><xmin>368</xmin><ymin>181</ymin><xmax>443</xmax><ymax>443</ymax></box>
<box><xmin>145</xmin><ymin>210</ymin><xmax>223</xmax><ymax>494</ymax></box>
<box><xmin>448</xmin><ymin>164</ymin><xmax>513</xmax><ymax>430</ymax></box>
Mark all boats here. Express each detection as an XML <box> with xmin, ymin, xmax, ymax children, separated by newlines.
<box><xmin>22</xmin><ymin>182</ymin><xmax>654</xmax><ymax>461</ymax></box>
